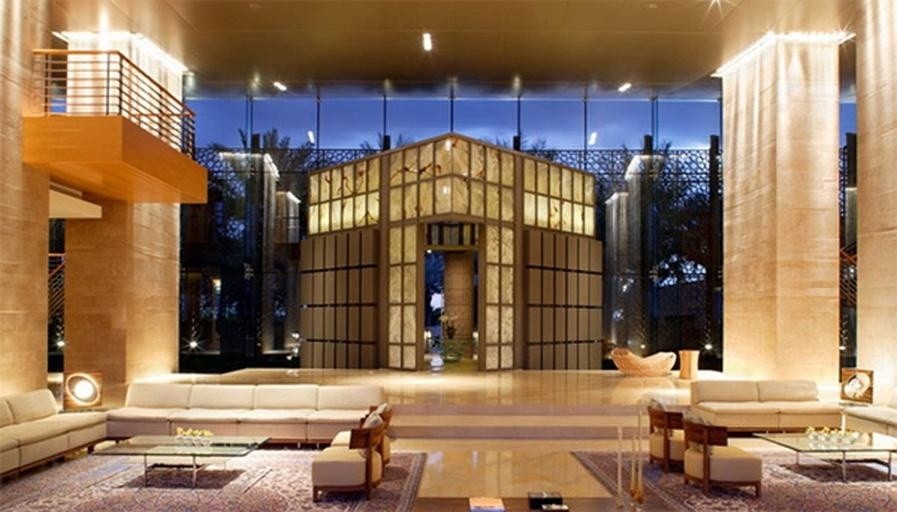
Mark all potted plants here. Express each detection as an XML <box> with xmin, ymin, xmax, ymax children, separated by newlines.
<box><xmin>441</xmin><ymin>340</ymin><xmax>465</xmax><ymax>362</ymax></box>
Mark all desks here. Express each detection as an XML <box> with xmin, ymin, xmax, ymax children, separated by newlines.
<box><xmin>410</xmin><ymin>496</ymin><xmax>669</xmax><ymax>512</ymax></box>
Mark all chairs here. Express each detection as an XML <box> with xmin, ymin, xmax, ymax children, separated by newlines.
<box><xmin>681</xmin><ymin>418</ymin><xmax>763</xmax><ymax>498</ymax></box>
<box><xmin>312</xmin><ymin>415</ymin><xmax>384</xmax><ymax>503</ymax></box>
<box><xmin>611</xmin><ymin>348</ymin><xmax>676</xmax><ymax>376</ymax></box>
<box><xmin>647</xmin><ymin>406</ymin><xmax>689</xmax><ymax>472</ymax></box>
<box><xmin>331</xmin><ymin>402</ymin><xmax>393</xmax><ymax>477</ymax></box>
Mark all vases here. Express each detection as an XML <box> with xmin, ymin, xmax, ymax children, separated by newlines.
<box><xmin>446</xmin><ymin>329</ymin><xmax>456</xmax><ymax>339</ymax></box>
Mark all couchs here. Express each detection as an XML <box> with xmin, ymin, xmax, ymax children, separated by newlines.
<box><xmin>844</xmin><ymin>386</ymin><xmax>897</xmax><ymax>438</ymax></box>
<box><xmin>106</xmin><ymin>384</ymin><xmax>388</xmax><ymax>449</ymax></box>
<box><xmin>0</xmin><ymin>388</ymin><xmax>107</xmax><ymax>481</ymax></box>
<box><xmin>691</xmin><ymin>380</ymin><xmax>842</xmax><ymax>434</ymax></box>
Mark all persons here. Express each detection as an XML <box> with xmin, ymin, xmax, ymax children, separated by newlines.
<box><xmin>428</xmin><ymin>281</ymin><xmax>443</xmax><ymax>347</ymax></box>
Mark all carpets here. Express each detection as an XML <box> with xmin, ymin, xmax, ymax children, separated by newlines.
<box><xmin>0</xmin><ymin>444</ymin><xmax>427</xmax><ymax>512</ymax></box>
<box><xmin>569</xmin><ymin>449</ymin><xmax>897</xmax><ymax>512</ymax></box>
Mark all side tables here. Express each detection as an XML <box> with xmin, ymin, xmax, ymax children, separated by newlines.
<box><xmin>679</xmin><ymin>350</ymin><xmax>700</xmax><ymax>379</ymax></box>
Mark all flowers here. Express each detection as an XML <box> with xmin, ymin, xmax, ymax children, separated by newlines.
<box><xmin>438</xmin><ymin>315</ymin><xmax>458</xmax><ymax>328</ymax></box>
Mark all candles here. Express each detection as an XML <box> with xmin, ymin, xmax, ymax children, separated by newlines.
<box><xmin>638</xmin><ymin>410</ymin><xmax>643</xmax><ymax>490</ymax></box>
<box><xmin>617</xmin><ymin>426</ymin><xmax>622</xmax><ymax>495</ymax></box>
<box><xmin>631</xmin><ymin>435</ymin><xmax>636</xmax><ymax>490</ymax></box>
